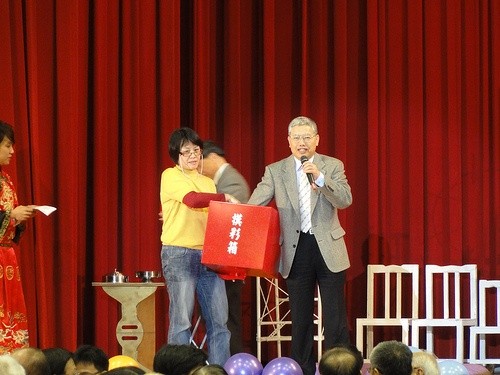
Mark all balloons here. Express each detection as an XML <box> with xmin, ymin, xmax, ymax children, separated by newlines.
<box><xmin>224</xmin><ymin>353</ymin><xmax>263</xmax><ymax>375</ymax></box>
<box><xmin>464</xmin><ymin>363</ymin><xmax>491</xmax><ymax>375</ymax></box>
<box><xmin>439</xmin><ymin>359</ymin><xmax>468</xmax><ymax>375</ymax></box>
<box><xmin>262</xmin><ymin>357</ymin><xmax>303</xmax><ymax>375</ymax></box>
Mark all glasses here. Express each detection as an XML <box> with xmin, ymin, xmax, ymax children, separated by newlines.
<box><xmin>180</xmin><ymin>149</ymin><xmax>203</xmax><ymax>157</ymax></box>
<box><xmin>290</xmin><ymin>133</ymin><xmax>318</xmax><ymax>142</ymax></box>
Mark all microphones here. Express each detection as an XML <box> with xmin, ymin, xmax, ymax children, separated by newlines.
<box><xmin>300</xmin><ymin>155</ymin><xmax>316</xmax><ymax>189</ymax></box>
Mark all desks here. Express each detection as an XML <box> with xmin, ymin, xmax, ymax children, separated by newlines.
<box><xmin>92</xmin><ymin>282</ymin><xmax>167</xmax><ymax>362</ymax></box>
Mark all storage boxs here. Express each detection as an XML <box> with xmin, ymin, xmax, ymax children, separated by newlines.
<box><xmin>200</xmin><ymin>200</ymin><xmax>280</xmax><ymax>280</ymax></box>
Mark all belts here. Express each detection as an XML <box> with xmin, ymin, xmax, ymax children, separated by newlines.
<box><xmin>300</xmin><ymin>231</ymin><xmax>313</xmax><ymax>235</ymax></box>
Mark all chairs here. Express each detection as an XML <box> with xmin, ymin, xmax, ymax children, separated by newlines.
<box><xmin>356</xmin><ymin>264</ymin><xmax>500</xmax><ymax>367</ymax></box>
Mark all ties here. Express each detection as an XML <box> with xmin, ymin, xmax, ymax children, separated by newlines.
<box><xmin>298</xmin><ymin>167</ymin><xmax>312</xmax><ymax>233</ymax></box>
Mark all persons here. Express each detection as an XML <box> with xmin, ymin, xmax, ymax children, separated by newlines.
<box><xmin>247</xmin><ymin>118</ymin><xmax>353</xmax><ymax>375</ymax></box>
<box><xmin>0</xmin><ymin>344</ymin><xmax>228</xmax><ymax>375</ymax></box>
<box><xmin>160</xmin><ymin>127</ymin><xmax>242</xmax><ymax>368</ymax></box>
<box><xmin>318</xmin><ymin>340</ymin><xmax>440</xmax><ymax>375</ymax></box>
<box><xmin>0</xmin><ymin>120</ymin><xmax>36</xmax><ymax>356</ymax></box>
<box><xmin>158</xmin><ymin>141</ymin><xmax>251</xmax><ymax>356</ymax></box>
